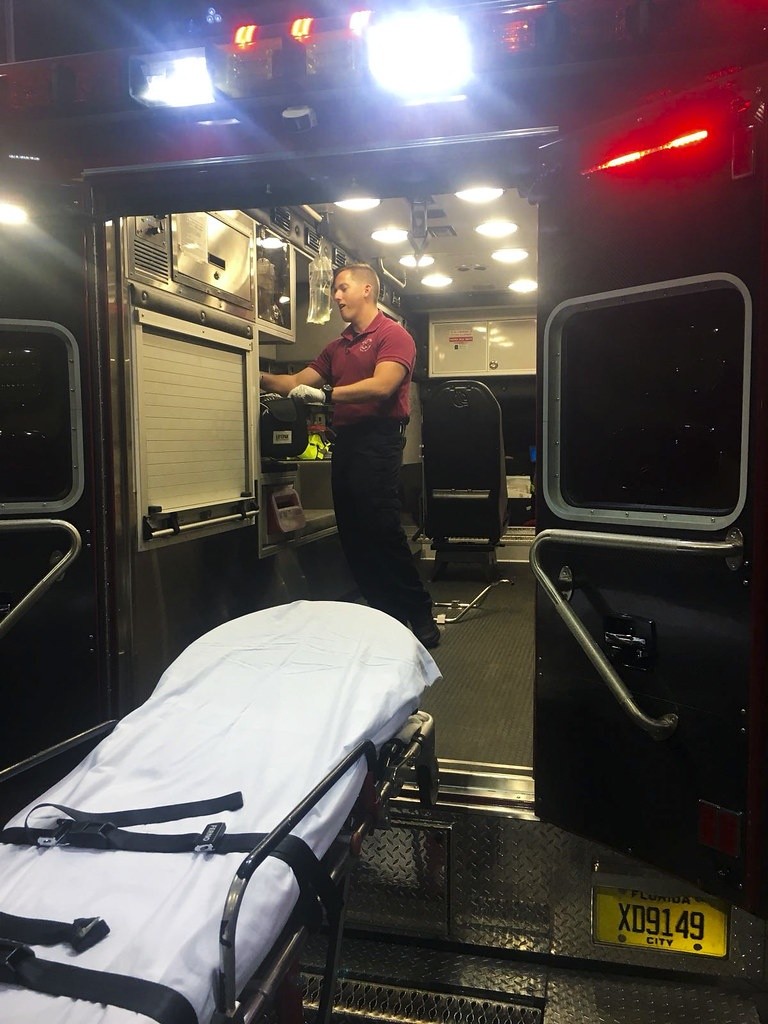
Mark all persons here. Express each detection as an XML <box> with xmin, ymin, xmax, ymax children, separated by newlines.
<box><xmin>258</xmin><ymin>265</ymin><xmax>444</xmax><ymax>648</ymax></box>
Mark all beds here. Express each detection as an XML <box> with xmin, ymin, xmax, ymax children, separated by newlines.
<box><xmin>0</xmin><ymin>597</ymin><xmax>444</xmax><ymax>1024</ymax></box>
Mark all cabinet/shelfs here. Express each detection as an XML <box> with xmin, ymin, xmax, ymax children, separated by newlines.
<box><xmin>429</xmin><ymin>312</ymin><xmax>536</xmax><ymax>376</ymax></box>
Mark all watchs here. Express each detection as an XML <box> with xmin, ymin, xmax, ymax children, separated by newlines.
<box><xmin>322</xmin><ymin>383</ymin><xmax>334</xmax><ymax>403</ymax></box>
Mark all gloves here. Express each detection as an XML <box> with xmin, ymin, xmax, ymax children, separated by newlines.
<box><xmin>287</xmin><ymin>384</ymin><xmax>325</xmax><ymax>404</ymax></box>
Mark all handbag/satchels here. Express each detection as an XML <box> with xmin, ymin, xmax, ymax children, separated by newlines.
<box><xmin>260</xmin><ymin>398</ymin><xmax>310</xmax><ymax>456</ymax></box>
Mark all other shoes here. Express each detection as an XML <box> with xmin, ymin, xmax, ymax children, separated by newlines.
<box><xmin>414</xmin><ymin>621</ymin><xmax>441</xmax><ymax>646</ymax></box>
